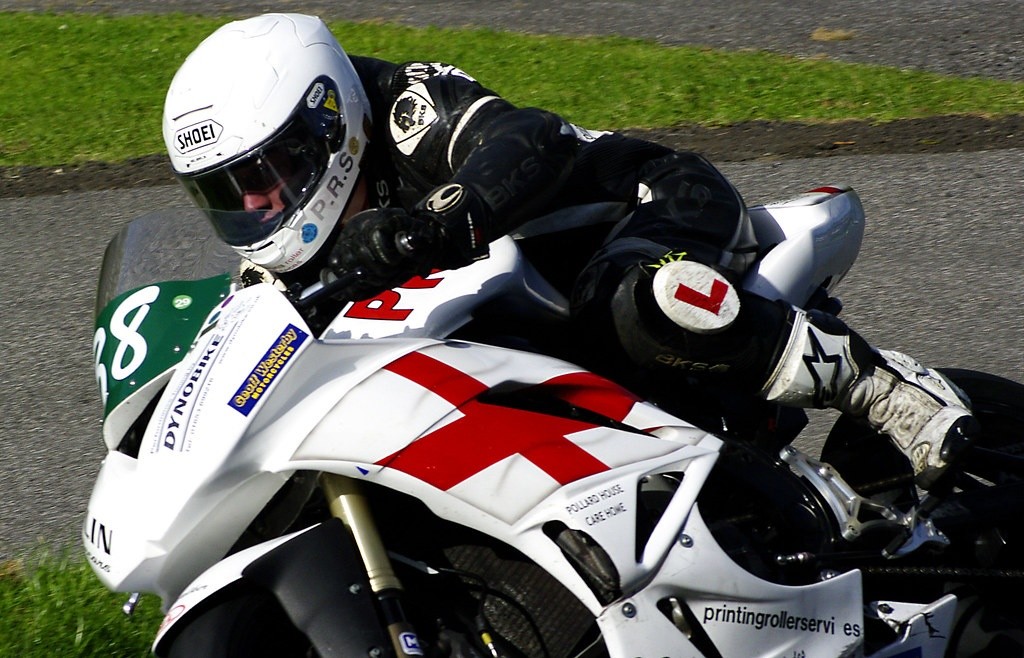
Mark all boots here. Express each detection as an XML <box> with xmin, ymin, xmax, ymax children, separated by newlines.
<box><xmin>746</xmin><ymin>300</ymin><xmax>974</xmax><ymax>494</ymax></box>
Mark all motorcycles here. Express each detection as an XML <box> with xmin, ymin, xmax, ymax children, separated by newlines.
<box><xmin>79</xmin><ymin>182</ymin><xmax>1024</xmax><ymax>658</ymax></box>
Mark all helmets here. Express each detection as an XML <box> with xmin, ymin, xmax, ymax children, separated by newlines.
<box><xmin>162</xmin><ymin>11</ymin><xmax>374</xmax><ymax>272</ymax></box>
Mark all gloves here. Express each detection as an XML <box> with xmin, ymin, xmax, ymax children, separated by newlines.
<box><xmin>329</xmin><ymin>207</ymin><xmax>450</xmax><ymax>298</ymax></box>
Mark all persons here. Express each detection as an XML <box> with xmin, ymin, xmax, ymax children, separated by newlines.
<box><xmin>160</xmin><ymin>13</ymin><xmax>975</xmax><ymax>490</ymax></box>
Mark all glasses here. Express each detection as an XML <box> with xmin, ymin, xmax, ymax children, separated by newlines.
<box><xmin>223</xmin><ymin>138</ymin><xmax>306</xmax><ymax>199</ymax></box>
